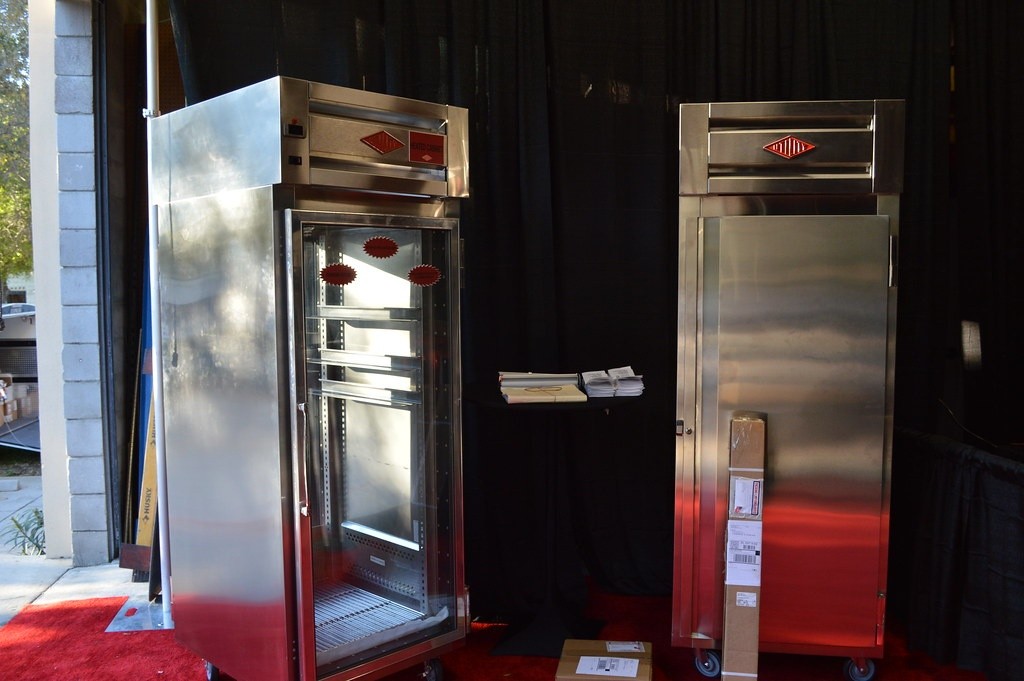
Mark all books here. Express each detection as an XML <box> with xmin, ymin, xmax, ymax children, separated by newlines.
<box><xmin>500</xmin><ymin>384</ymin><xmax>587</xmax><ymax>404</ymax></box>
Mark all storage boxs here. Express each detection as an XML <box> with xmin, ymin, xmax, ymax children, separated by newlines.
<box><xmin>556</xmin><ymin>639</ymin><xmax>652</xmax><ymax>681</ymax></box>
<box><xmin>0</xmin><ymin>374</ymin><xmax>18</xmax><ymax>427</ymax></box>
